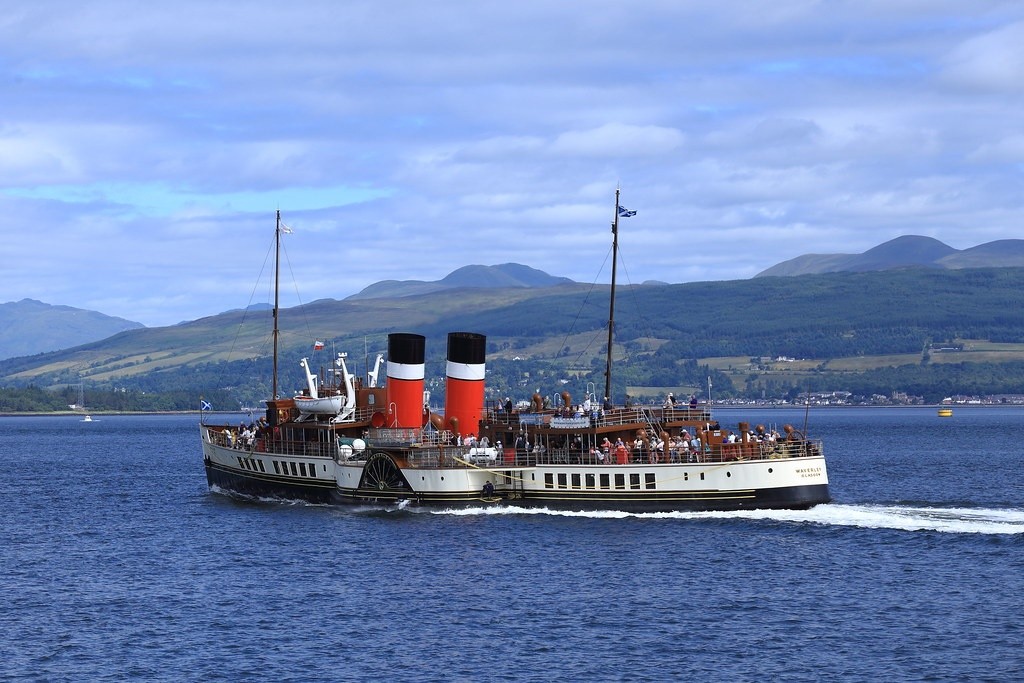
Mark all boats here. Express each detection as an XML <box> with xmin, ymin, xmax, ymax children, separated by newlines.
<box><xmin>293</xmin><ymin>356</ymin><xmax>347</xmax><ymax>414</ymax></box>
<box><xmin>938</xmin><ymin>408</ymin><xmax>953</xmax><ymax>417</ymax></box>
<box><xmin>79</xmin><ymin>414</ymin><xmax>101</xmax><ymax>423</ymax></box>
<box><xmin>195</xmin><ymin>185</ymin><xmax>834</xmax><ymax>514</ymax></box>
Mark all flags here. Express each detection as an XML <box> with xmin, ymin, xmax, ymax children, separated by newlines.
<box><xmin>314</xmin><ymin>340</ymin><xmax>324</xmax><ymax>350</ymax></box>
<box><xmin>201</xmin><ymin>399</ymin><xmax>213</xmax><ymax>411</ymax></box>
<box><xmin>281</xmin><ymin>224</ymin><xmax>292</xmax><ymax>233</ymax></box>
<box><xmin>619</xmin><ymin>205</ymin><xmax>636</xmax><ymax>217</ymax></box>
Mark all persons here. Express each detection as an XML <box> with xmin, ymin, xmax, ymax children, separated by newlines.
<box><xmin>648</xmin><ymin>429</ymin><xmax>702</xmax><ymax>463</ymax></box>
<box><xmin>464</xmin><ymin>433</ymin><xmax>480</xmax><ymax>448</ymax></box>
<box><xmin>479</xmin><ymin>436</ymin><xmax>490</xmax><ymax>448</ymax></box>
<box><xmin>504</xmin><ymin>397</ymin><xmax>512</xmax><ymax>414</ymax></box>
<box><xmin>634</xmin><ymin>436</ymin><xmax>642</xmax><ymax>462</ymax></box>
<box><xmin>689</xmin><ymin>395</ymin><xmax>697</xmax><ymax>409</ymax></box>
<box><xmin>668</xmin><ymin>393</ymin><xmax>679</xmax><ymax>408</ymax></box>
<box><xmin>496</xmin><ymin>398</ymin><xmax>503</xmax><ymax>414</ymax></box>
<box><xmin>721</xmin><ymin>430</ymin><xmax>780</xmax><ymax>447</ymax></box>
<box><xmin>219</xmin><ymin>416</ymin><xmax>269</xmax><ymax>453</ymax></box>
<box><xmin>455</xmin><ymin>432</ymin><xmax>463</xmax><ymax>447</ymax></box>
<box><xmin>495</xmin><ymin>440</ymin><xmax>504</xmax><ymax>466</ymax></box>
<box><xmin>601</xmin><ymin>437</ymin><xmax>611</xmax><ymax>464</ymax></box>
<box><xmin>555</xmin><ymin>406</ymin><xmax>575</xmax><ymax>420</ymax></box>
<box><xmin>590</xmin><ymin>445</ymin><xmax>605</xmax><ymax>461</ymax></box>
<box><xmin>615</xmin><ymin>437</ymin><xmax>626</xmax><ymax>465</ymax></box>
